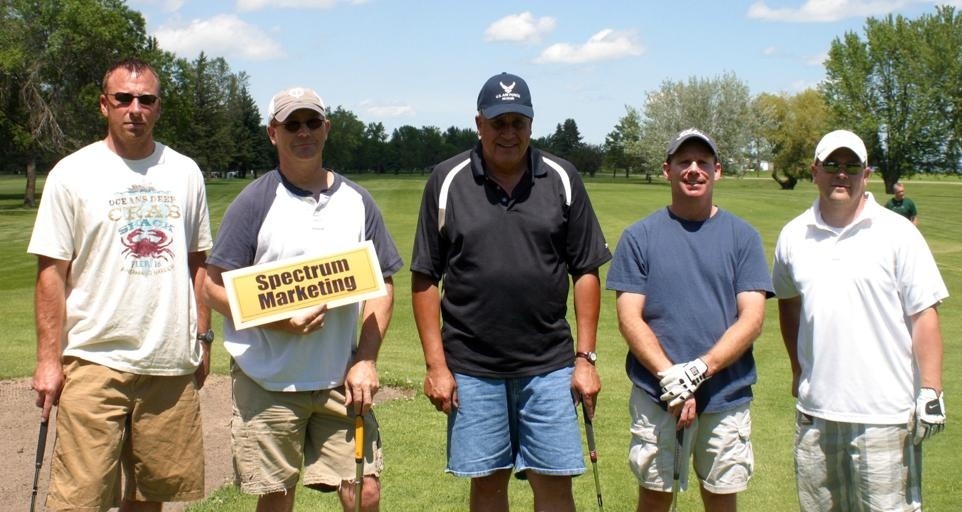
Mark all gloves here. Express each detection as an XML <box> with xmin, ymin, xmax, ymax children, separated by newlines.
<box><xmin>912</xmin><ymin>386</ymin><xmax>947</xmax><ymax>447</ymax></box>
<box><xmin>657</xmin><ymin>357</ymin><xmax>713</xmax><ymax>409</ymax></box>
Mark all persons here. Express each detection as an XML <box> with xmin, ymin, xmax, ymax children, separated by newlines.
<box><xmin>603</xmin><ymin>127</ymin><xmax>776</xmax><ymax>512</ymax></box>
<box><xmin>25</xmin><ymin>57</ymin><xmax>216</xmax><ymax>512</ymax></box>
<box><xmin>202</xmin><ymin>85</ymin><xmax>403</xmax><ymax>512</ymax></box>
<box><xmin>406</xmin><ymin>71</ymin><xmax>614</xmax><ymax>512</ymax></box>
<box><xmin>884</xmin><ymin>180</ymin><xmax>919</xmax><ymax>226</ymax></box>
<box><xmin>774</xmin><ymin>128</ymin><xmax>952</xmax><ymax>511</ymax></box>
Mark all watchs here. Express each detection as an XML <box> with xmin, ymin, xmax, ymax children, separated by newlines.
<box><xmin>199</xmin><ymin>330</ymin><xmax>215</xmax><ymax>347</ymax></box>
<box><xmin>575</xmin><ymin>350</ymin><xmax>598</xmax><ymax>365</ymax></box>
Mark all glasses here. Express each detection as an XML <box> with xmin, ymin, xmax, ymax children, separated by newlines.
<box><xmin>101</xmin><ymin>90</ymin><xmax>160</xmax><ymax>105</ymax></box>
<box><xmin>816</xmin><ymin>159</ymin><xmax>866</xmax><ymax>177</ymax></box>
<box><xmin>274</xmin><ymin>116</ymin><xmax>327</xmax><ymax>133</ymax></box>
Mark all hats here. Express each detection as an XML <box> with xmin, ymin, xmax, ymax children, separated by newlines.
<box><xmin>477</xmin><ymin>74</ymin><xmax>534</xmax><ymax>121</ymax></box>
<box><xmin>269</xmin><ymin>85</ymin><xmax>326</xmax><ymax>127</ymax></box>
<box><xmin>665</xmin><ymin>127</ymin><xmax>721</xmax><ymax>165</ymax></box>
<box><xmin>814</xmin><ymin>130</ymin><xmax>869</xmax><ymax>167</ymax></box>
